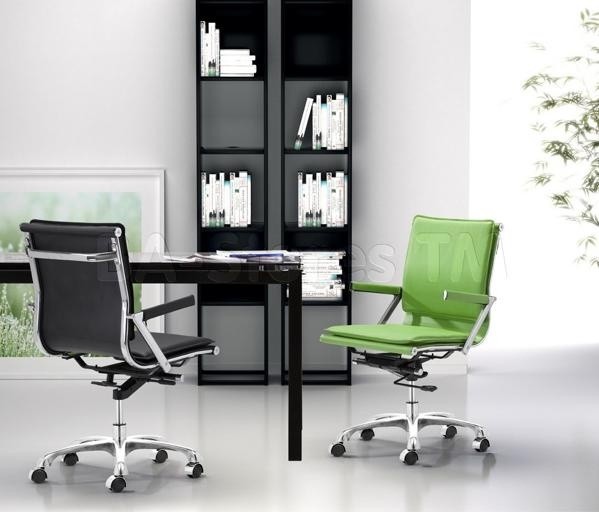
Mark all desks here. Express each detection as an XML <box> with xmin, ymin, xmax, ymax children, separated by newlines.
<box><xmin>0</xmin><ymin>251</ymin><xmax>303</xmax><ymax>461</ymax></box>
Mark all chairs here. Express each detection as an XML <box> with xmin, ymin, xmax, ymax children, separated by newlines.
<box><xmin>19</xmin><ymin>219</ymin><xmax>220</xmax><ymax>494</ymax></box>
<box><xmin>319</xmin><ymin>214</ymin><xmax>503</xmax><ymax>466</ymax></box>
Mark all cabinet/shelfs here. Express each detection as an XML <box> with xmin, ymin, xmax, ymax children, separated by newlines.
<box><xmin>195</xmin><ymin>0</ymin><xmax>269</xmax><ymax>386</ymax></box>
<box><xmin>280</xmin><ymin>0</ymin><xmax>352</xmax><ymax>386</ymax></box>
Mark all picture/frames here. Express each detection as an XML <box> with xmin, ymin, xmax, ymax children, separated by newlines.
<box><xmin>0</xmin><ymin>166</ymin><xmax>165</xmax><ymax>381</ymax></box>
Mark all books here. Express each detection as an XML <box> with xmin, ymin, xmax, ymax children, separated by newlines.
<box><xmin>199</xmin><ymin>22</ymin><xmax>256</xmax><ymax>76</ymax></box>
<box><xmin>200</xmin><ymin>167</ymin><xmax>253</xmax><ymax>231</ymax></box>
<box><xmin>165</xmin><ymin>243</ymin><xmax>286</xmax><ymax>266</ymax></box>
<box><xmin>285</xmin><ymin>88</ymin><xmax>350</xmax><ymax>301</ymax></box>
